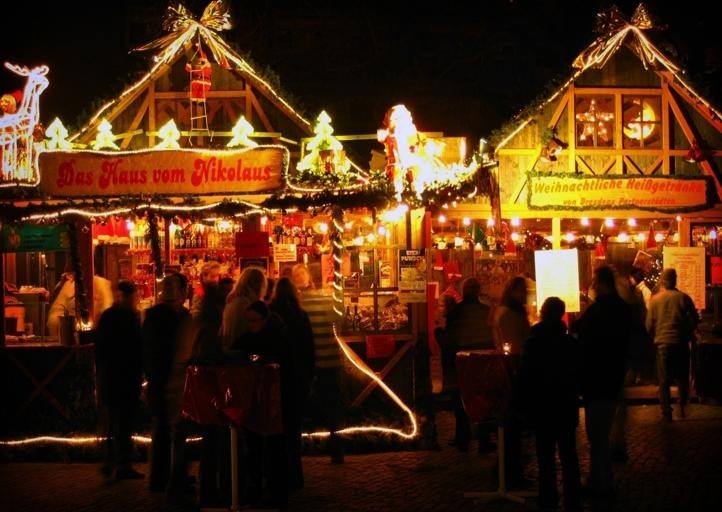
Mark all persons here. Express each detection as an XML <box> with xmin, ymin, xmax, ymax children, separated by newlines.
<box><xmin>433</xmin><ymin>256</ymin><xmax>700</xmax><ymax>512</ymax></box>
<box><xmin>47</xmin><ymin>257</ymin><xmax>115</xmax><ymax>337</ymax></box>
<box><xmin>92</xmin><ymin>264</ymin><xmax>319</xmax><ymax>512</ymax></box>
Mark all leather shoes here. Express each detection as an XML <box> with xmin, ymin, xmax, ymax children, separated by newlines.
<box><xmin>117</xmin><ymin>467</ymin><xmax>145</xmax><ymax>479</ymax></box>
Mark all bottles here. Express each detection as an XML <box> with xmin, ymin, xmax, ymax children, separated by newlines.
<box><xmin>346</xmin><ymin>268</ymin><xmax>363</xmax><ymax>286</ymax></box>
<box><xmin>342</xmin><ymin>305</ymin><xmax>352</xmax><ymax>335</ymax></box>
<box><xmin>57</xmin><ymin>309</ymin><xmax>75</xmax><ymax>344</ymax></box>
<box><xmin>103</xmin><ymin>223</ymin><xmax>316</xmax><ymax>251</ymax></box>
<box><xmin>353</xmin><ymin>305</ymin><xmax>360</xmax><ymax>335</ymax></box>
<box><xmin>25</xmin><ymin>323</ymin><xmax>34</xmax><ymax>335</ymax></box>
